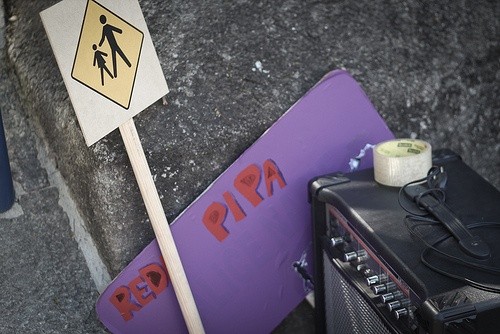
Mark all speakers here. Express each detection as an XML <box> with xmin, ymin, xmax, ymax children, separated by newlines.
<box><xmin>308</xmin><ymin>149</ymin><xmax>500</xmax><ymax>334</ymax></box>
